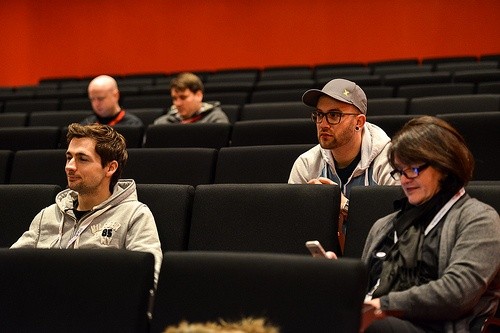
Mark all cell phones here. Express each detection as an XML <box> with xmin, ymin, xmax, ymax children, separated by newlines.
<box><xmin>306</xmin><ymin>241</ymin><xmax>326</xmax><ymax>257</ymax></box>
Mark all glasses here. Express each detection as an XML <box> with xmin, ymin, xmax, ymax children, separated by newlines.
<box><xmin>310</xmin><ymin>110</ymin><xmax>360</xmax><ymax>124</ymax></box>
<box><xmin>390</xmin><ymin>163</ymin><xmax>430</xmax><ymax>181</ymax></box>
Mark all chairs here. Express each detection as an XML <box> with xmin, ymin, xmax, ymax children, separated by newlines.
<box><xmin>0</xmin><ymin>50</ymin><xmax>499</xmax><ymax>333</ymax></box>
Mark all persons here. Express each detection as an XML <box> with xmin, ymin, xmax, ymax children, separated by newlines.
<box><xmin>10</xmin><ymin>123</ymin><xmax>162</xmax><ymax>319</ymax></box>
<box><xmin>311</xmin><ymin>115</ymin><xmax>500</xmax><ymax>333</ymax></box>
<box><xmin>287</xmin><ymin>78</ymin><xmax>401</xmax><ymax>255</ymax></box>
<box><xmin>80</xmin><ymin>75</ymin><xmax>142</xmax><ymax>127</ymax></box>
<box><xmin>152</xmin><ymin>72</ymin><xmax>229</xmax><ymax>125</ymax></box>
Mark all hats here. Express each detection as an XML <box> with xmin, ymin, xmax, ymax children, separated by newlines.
<box><xmin>302</xmin><ymin>79</ymin><xmax>368</xmax><ymax>115</ymax></box>
